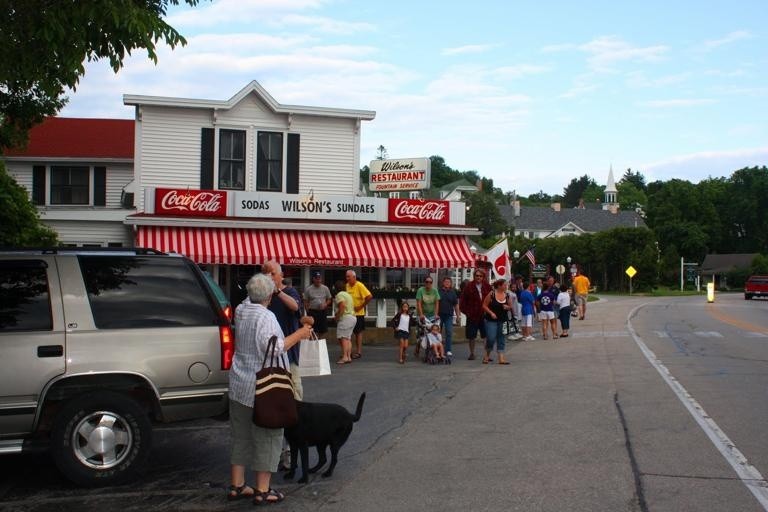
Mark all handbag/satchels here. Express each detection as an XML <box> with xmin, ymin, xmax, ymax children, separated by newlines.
<box><xmin>298</xmin><ymin>338</ymin><xmax>331</xmax><ymax>378</ymax></box>
<box><xmin>253</xmin><ymin>367</ymin><xmax>298</xmax><ymax>429</ymax></box>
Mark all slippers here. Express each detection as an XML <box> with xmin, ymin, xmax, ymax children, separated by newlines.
<box><xmin>336</xmin><ymin>359</ymin><xmax>352</xmax><ymax>363</ymax></box>
<box><xmin>483</xmin><ymin>358</ymin><xmax>509</xmax><ymax>364</ymax></box>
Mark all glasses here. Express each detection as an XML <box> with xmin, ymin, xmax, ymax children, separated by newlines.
<box><xmin>476</xmin><ymin>275</ymin><xmax>483</xmax><ymax>277</ymax></box>
<box><xmin>425</xmin><ymin>281</ymin><xmax>432</xmax><ymax>284</ymax></box>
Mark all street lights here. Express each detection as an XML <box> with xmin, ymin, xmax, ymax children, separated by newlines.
<box><xmin>513</xmin><ymin>250</ymin><xmax>520</xmax><ymax>278</ymax></box>
<box><xmin>468</xmin><ymin>244</ymin><xmax>476</xmax><ymax>257</ymax></box>
<box><xmin>565</xmin><ymin>255</ymin><xmax>573</xmax><ymax>287</ymax></box>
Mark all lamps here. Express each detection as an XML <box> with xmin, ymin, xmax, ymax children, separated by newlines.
<box><xmin>186</xmin><ymin>184</ymin><xmax>190</xmax><ymax>197</ymax></box>
<box><xmin>308</xmin><ymin>189</ymin><xmax>314</xmax><ymax>200</ymax></box>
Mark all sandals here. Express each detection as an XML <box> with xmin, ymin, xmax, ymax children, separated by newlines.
<box><xmin>352</xmin><ymin>353</ymin><xmax>361</xmax><ymax>359</ymax></box>
<box><xmin>253</xmin><ymin>487</ymin><xmax>289</xmax><ymax>505</ymax></box>
<box><xmin>228</xmin><ymin>484</ymin><xmax>256</xmax><ymax>502</ymax></box>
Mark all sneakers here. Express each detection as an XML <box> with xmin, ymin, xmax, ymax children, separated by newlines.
<box><xmin>544</xmin><ymin>333</ymin><xmax>568</xmax><ymax>340</ymax></box>
<box><xmin>447</xmin><ymin>351</ymin><xmax>452</xmax><ymax>356</ymax></box>
<box><xmin>522</xmin><ymin>335</ymin><xmax>535</xmax><ymax>341</ymax></box>
<box><xmin>468</xmin><ymin>353</ymin><xmax>474</xmax><ymax>360</ymax></box>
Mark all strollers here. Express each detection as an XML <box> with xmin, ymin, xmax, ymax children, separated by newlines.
<box><xmin>414</xmin><ymin>314</ymin><xmax>453</xmax><ymax>364</ymax></box>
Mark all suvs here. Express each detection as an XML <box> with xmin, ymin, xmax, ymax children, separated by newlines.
<box><xmin>0</xmin><ymin>241</ymin><xmax>239</xmax><ymax>483</ymax></box>
<box><xmin>169</xmin><ymin>250</ymin><xmax>237</xmax><ymax>331</ymax></box>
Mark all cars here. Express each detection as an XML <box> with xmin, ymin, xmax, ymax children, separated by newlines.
<box><xmin>744</xmin><ymin>275</ymin><xmax>768</xmax><ymax>300</ymax></box>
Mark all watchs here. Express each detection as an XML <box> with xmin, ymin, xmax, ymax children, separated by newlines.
<box><xmin>275</xmin><ymin>288</ymin><xmax>281</xmax><ymax>294</ymax></box>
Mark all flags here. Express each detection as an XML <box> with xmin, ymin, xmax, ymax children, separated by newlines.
<box><xmin>526</xmin><ymin>248</ymin><xmax>536</xmax><ymax>268</ymax></box>
<box><xmin>486</xmin><ymin>238</ymin><xmax>512</xmax><ymax>281</ymax></box>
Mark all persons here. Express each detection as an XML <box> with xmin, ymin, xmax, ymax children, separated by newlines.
<box><xmin>346</xmin><ymin>269</ymin><xmax>373</xmax><ymax>359</ymax></box>
<box><xmin>279</xmin><ymin>265</ymin><xmax>292</xmax><ymax>287</ymax></box>
<box><xmin>302</xmin><ymin>272</ymin><xmax>333</xmax><ymax>339</ymax></box>
<box><xmin>332</xmin><ymin>279</ymin><xmax>358</xmax><ymax>364</ymax></box>
<box><xmin>261</xmin><ymin>262</ymin><xmax>304</xmax><ymax>471</ymax></box>
<box><xmin>226</xmin><ymin>274</ymin><xmax>312</xmax><ymax>505</ymax></box>
<box><xmin>391</xmin><ymin>263</ymin><xmax>592</xmax><ymax>364</ymax></box>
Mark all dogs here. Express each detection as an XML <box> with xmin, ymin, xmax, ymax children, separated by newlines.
<box><xmin>284</xmin><ymin>392</ymin><xmax>365</xmax><ymax>484</ymax></box>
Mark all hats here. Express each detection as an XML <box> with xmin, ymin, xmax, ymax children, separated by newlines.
<box><xmin>313</xmin><ymin>272</ymin><xmax>321</xmax><ymax>276</ymax></box>
